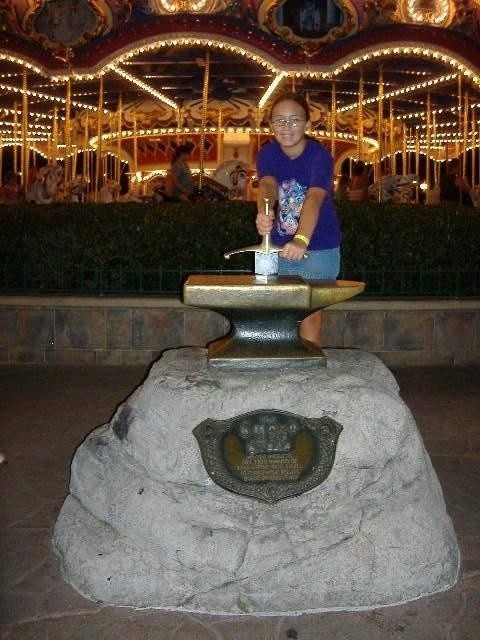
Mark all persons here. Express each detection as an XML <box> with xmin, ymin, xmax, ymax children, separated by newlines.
<box><xmin>1</xmin><ymin>161</ymin><xmax>476</xmax><ymax>208</ymax></box>
<box><xmin>254</xmin><ymin>90</ymin><xmax>343</xmax><ymax>349</ymax></box>
<box><xmin>337</xmin><ymin>156</ymin><xmax>356</xmax><ymax>193</ymax></box>
<box><xmin>168</xmin><ymin>145</ymin><xmax>194</xmax><ymax>203</ymax></box>
<box><xmin>444</xmin><ymin>154</ymin><xmax>474</xmax><ymax>210</ymax></box>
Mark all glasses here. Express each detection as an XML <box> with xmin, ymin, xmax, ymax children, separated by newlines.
<box><xmin>271</xmin><ymin>118</ymin><xmax>306</xmax><ymax>127</ymax></box>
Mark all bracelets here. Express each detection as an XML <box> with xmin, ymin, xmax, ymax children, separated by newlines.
<box><xmin>292</xmin><ymin>234</ymin><xmax>311</xmax><ymax>247</ymax></box>
